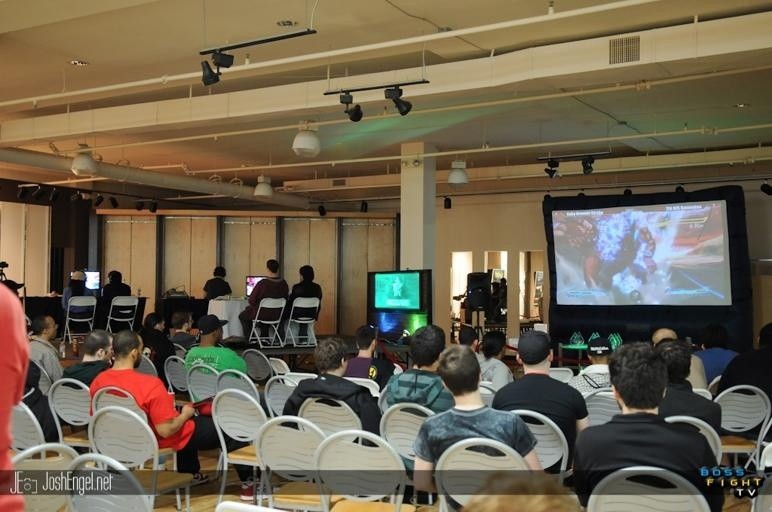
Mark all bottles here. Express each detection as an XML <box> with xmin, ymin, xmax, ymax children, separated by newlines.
<box><xmin>58</xmin><ymin>339</ymin><xmax>66</xmax><ymax>358</ymax></box>
<box><xmin>137</xmin><ymin>288</ymin><xmax>142</xmax><ymax>297</ymax></box>
<box><xmin>72</xmin><ymin>338</ymin><xmax>80</xmax><ymax>356</ymax></box>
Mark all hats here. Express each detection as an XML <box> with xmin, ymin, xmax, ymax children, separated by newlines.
<box><xmin>197</xmin><ymin>313</ymin><xmax>229</xmax><ymax>336</ymax></box>
<box><xmin>652</xmin><ymin>327</ymin><xmax>679</xmax><ymax>345</ymax></box>
<box><xmin>70</xmin><ymin>270</ymin><xmax>87</xmax><ymax>282</ymax></box>
<box><xmin>585</xmin><ymin>336</ymin><xmax>614</xmax><ymax>357</ymax></box>
<box><xmin>518</xmin><ymin>328</ymin><xmax>553</xmax><ymax>365</ymax></box>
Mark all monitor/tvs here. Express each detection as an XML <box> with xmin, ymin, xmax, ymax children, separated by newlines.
<box><xmin>246</xmin><ymin>275</ymin><xmax>269</xmax><ymax>296</ymax></box>
<box><xmin>367</xmin><ymin>270</ymin><xmax>432</xmax><ymax>310</ymax></box>
<box><xmin>70</xmin><ymin>271</ymin><xmax>101</xmax><ymax>290</ymax></box>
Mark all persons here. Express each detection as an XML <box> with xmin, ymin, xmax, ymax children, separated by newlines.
<box><xmin>0</xmin><ymin>281</ymin><xmax>30</xmax><ymax>511</ymax></box>
<box><xmin>274</xmin><ymin>266</ymin><xmax>322</xmax><ymax>345</ymax></box>
<box><xmin>3</xmin><ymin>280</ymin><xmax>25</xmax><ymax>297</ymax></box>
<box><xmin>61</xmin><ymin>271</ymin><xmax>93</xmax><ymax>340</ymax></box>
<box><xmin>388</xmin><ymin>275</ymin><xmax>408</xmax><ymax>300</ymax></box>
<box><xmin>492</xmin><ymin>278</ymin><xmax>507</xmax><ymax>320</ymax></box>
<box><xmin>203</xmin><ymin>266</ymin><xmax>232</xmax><ymax>300</ymax></box>
<box><xmin>21</xmin><ymin>311</ymin><xmax>772</xmax><ymax>511</ymax></box>
<box><xmin>102</xmin><ymin>271</ymin><xmax>133</xmax><ymax>318</ymax></box>
<box><xmin>239</xmin><ymin>260</ymin><xmax>288</xmax><ymax>345</ymax></box>
<box><xmin>490</xmin><ymin>282</ymin><xmax>500</xmax><ymax>306</ymax></box>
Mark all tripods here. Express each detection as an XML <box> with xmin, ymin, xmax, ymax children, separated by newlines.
<box><xmin>473</xmin><ymin>311</ymin><xmax>484</xmax><ymax>343</ymax></box>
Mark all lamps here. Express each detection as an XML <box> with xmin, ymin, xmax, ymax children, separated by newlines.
<box><xmin>253</xmin><ymin>169</ymin><xmax>272</xmax><ymax>196</ymax></box>
<box><xmin>195</xmin><ymin>51</ymin><xmax>234</xmax><ymax>88</ymax></box>
<box><xmin>292</xmin><ymin>120</ymin><xmax>323</xmax><ymax>160</ymax></box>
<box><xmin>447</xmin><ymin>151</ymin><xmax>472</xmax><ymax>185</ymax></box>
<box><xmin>318</xmin><ymin>195</ymin><xmax>452</xmax><ymax>221</ymax></box>
<box><xmin>17</xmin><ymin>184</ymin><xmax>159</xmax><ymax>215</ymax></box>
<box><xmin>544</xmin><ymin>178</ymin><xmax>771</xmax><ymax>200</ymax></box>
<box><xmin>71</xmin><ymin>137</ymin><xmax>98</xmax><ymax>179</ymax></box>
<box><xmin>543</xmin><ymin>157</ymin><xmax>595</xmax><ymax>179</ymax></box>
<box><xmin>339</xmin><ymin>86</ymin><xmax>413</xmax><ymax>124</ymax></box>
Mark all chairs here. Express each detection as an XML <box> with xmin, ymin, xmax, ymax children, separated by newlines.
<box><xmin>1</xmin><ymin>282</ymin><xmax>771</xmax><ymax>511</ymax></box>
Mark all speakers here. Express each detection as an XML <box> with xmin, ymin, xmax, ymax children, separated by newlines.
<box><xmin>466</xmin><ymin>272</ymin><xmax>491</xmax><ymax>311</ymax></box>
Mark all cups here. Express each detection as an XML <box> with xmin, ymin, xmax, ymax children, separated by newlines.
<box><xmin>168</xmin><ymin>392</ymin><xmax>175</xmax><ymax>403</ymax></box>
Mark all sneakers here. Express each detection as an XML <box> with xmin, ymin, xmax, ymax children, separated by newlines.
<box><xmin>248</xmin><ymin>342</ymin><xmax>258</xmax><ymax>348</ymax></box>
<box><xmin>261</xmin><ymin>339</ymin><xmax>271</xmax><ymax>346</ymax></box>
<box><xmin>180</xmin><ymin>470</ymin><xmax>209</xmax><ymax>489</ymax></box>
<box><xmin>296</xmin><ymin>341</ymin><xmax>308</xmax><ymax>345</ymax></box>
<box><xmin>275</xmin><ymin>341</ymin><xmax>288</xmax><ymax>346</ymax></box>
<box><xmin>239</xmin><ymin>479</ymin><xmax>281</xmax><ymax>502</ymax></box>
<box><xmin>158</xmin><ymin>462</ymin><xmax>167</xmax><ymax>472</ymax></box>
